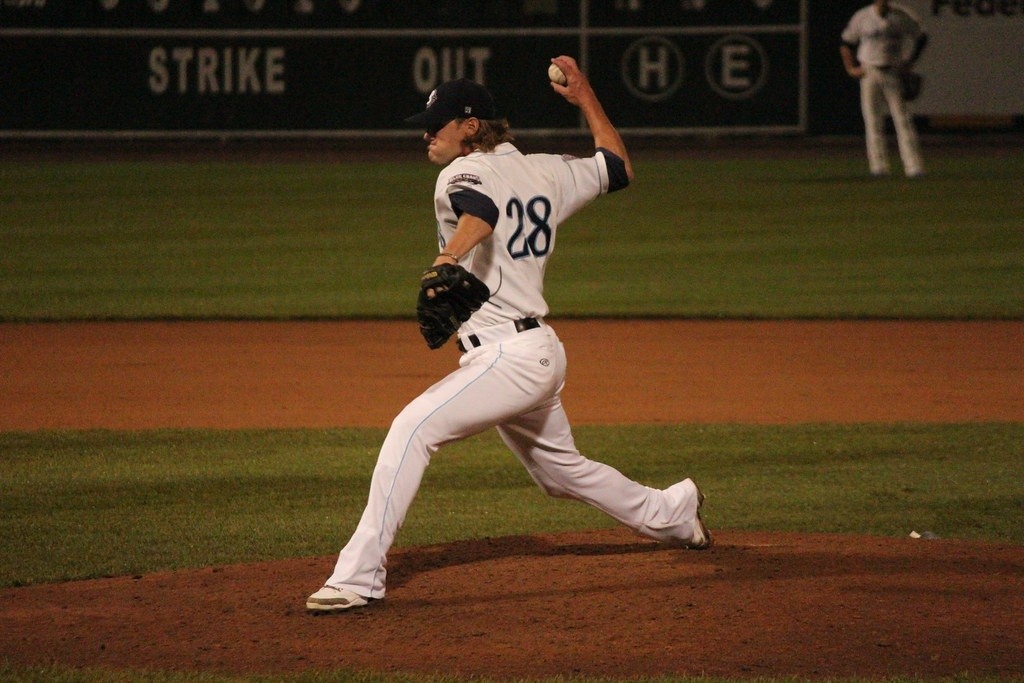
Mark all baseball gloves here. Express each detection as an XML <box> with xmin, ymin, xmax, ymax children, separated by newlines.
<box><xmin>416</xmin><ymin>263</ymin><xmax>490</xmax><ymax>351</ymax></box>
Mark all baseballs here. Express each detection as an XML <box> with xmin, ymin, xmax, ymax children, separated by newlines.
<box><xmin>548</xmin><ymin>63</ymin><xmax>567</xmax><ymax>85</ymax></box>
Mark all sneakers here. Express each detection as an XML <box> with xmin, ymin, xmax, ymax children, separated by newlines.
<box><xmin>305</xmin><ymin>584</ymin><xmax>386</xmax><ymax>612</ymax></box>
<box><xmin>665</xmin><ymin>477</ymin><xmax>714</xmax><ymax>551</ymax></box>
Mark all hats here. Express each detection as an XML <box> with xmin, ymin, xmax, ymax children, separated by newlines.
<box><xmin>405</xmin><ymin>79</ymin><xmax>496</xmax><ymax>127</ymax></box>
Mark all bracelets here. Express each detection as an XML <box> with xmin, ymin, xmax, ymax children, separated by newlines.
<box><xmin>438</xmin><ymin>252</ymin><xmax>459</xmax><ymax>262</ymax></box>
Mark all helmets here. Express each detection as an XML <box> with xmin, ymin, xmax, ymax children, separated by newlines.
<box><xmin>900</xmin><ymin>71</ymin><xmax>920</xmax><ymax>101</ymax></box>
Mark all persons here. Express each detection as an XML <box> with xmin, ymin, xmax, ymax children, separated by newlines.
<box><xmin>303</xmin><ymin>54</ymin><xmax>711</xmax><ymax>616</ymax></box>
<box><xmin>839</xmin><ymin>0</ymin><xmax>932</xmax><ymax>182</ymax></box>
